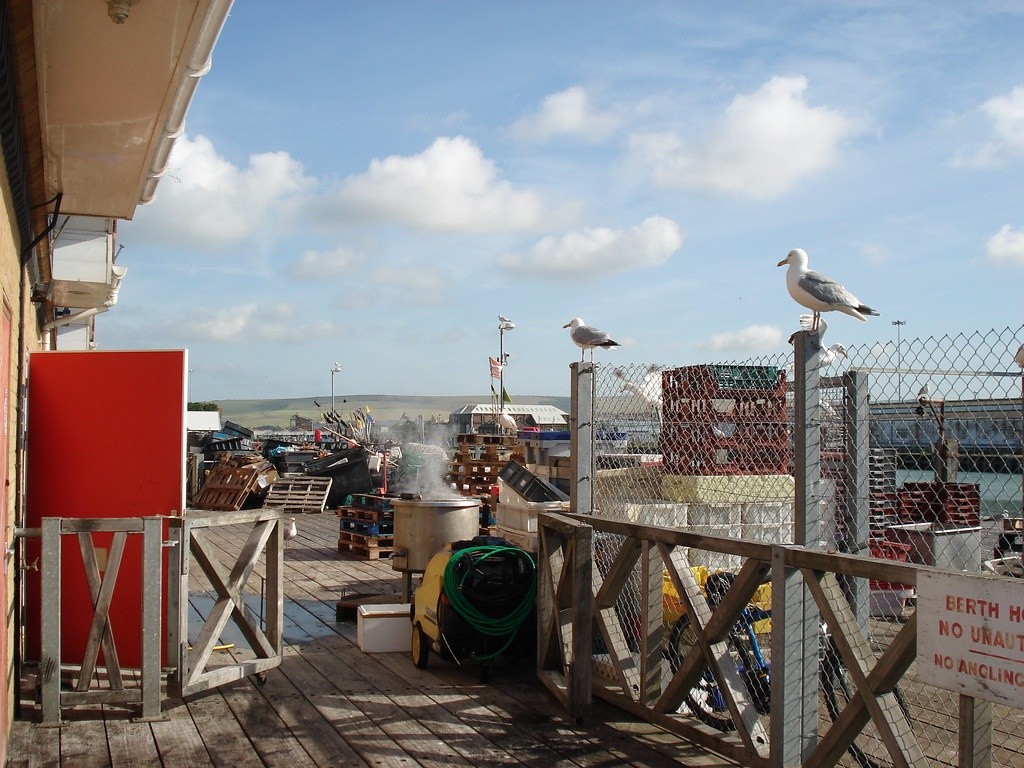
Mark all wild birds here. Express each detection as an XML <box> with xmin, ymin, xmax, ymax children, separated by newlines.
<box><xmin>788</xmin><ymin>315</ymin><xmax>848</xmax><ymax>375</ymax></box>
<box><xmin>284</xmin><ymin>517</ymin><xmax>297</xmax><ymax>550</ymax></box>
<box><xmin>620</xmin><ymin>379</ymin><xmax>666</xmax><ymax>423</ymax></box>
<box><xmin>776</xmin><ymin>248</ymin><xmax>880</xmax><ymax>330</ymax></box>
<box><xmin>917</xmin><ymin>383</ymin><xmax>931</xmax><ymax>397</ymax></box>
<box><xmin>499</xmin><ymin>409</ymin><xmax>519</xmax><ymax>432</ymax></box>
<box><xmin>562</xmin><ymin>318</ymin><xmax>619</xmax><ymax>360</ymax></box>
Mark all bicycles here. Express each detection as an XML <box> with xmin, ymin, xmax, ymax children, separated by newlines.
<box><xmin>668</xmin><ymin>544</ymin><xmax>912</xmax><ymax>768</ymax></box>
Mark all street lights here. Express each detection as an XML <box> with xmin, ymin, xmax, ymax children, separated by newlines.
<box><xmin>500</xmin><ymin>322</ymin><xmax>515</xmax><ymax>413</ymax></box>
<box><xmin>189</xmin><ymin>369</ymin><xmax>193</xmax><ymax>402</ymax></box>
<box><xmin>893</xmin><ymin>320</ymin><xmax>907</xmax><ymax>402</ymax></box>
<box><xmin>331</xmin><ymin>368</ymin><xmax>341</xmax><ymax>420</ymax></box>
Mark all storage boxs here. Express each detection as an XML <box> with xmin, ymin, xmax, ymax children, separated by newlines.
<box><xmin>666</xmin><ymin>366</ymin><xmax>983</xmax><ymax>618</ymax></box>
<box><xmin>492</xmin><ymin>461</ymin><xmax>573</xmax><ymax>535</ymax></box>
<box><xmin>355</xmin><ymin>602</ymin><xmax>416</xmax><ymax>653</ymax></box>
